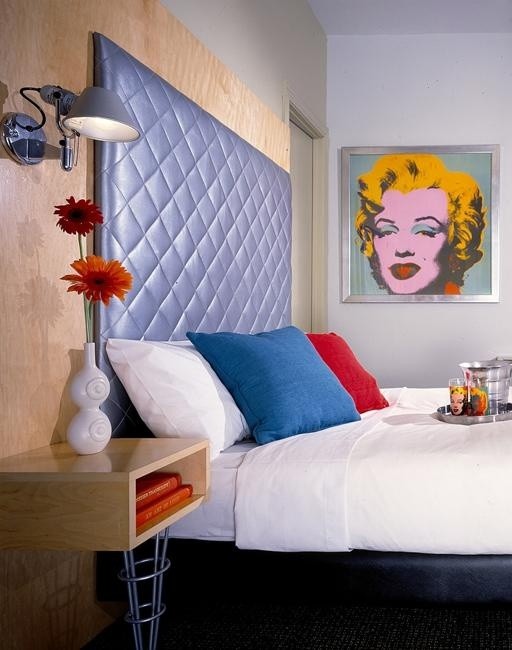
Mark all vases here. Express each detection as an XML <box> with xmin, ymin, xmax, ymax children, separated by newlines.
<box><xmin>66</xmin><ymin>344</ymin><xmax>111</xmax><ymax>456</ymax></box>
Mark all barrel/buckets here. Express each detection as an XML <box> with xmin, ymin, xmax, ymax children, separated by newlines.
<box><xmin>457</xmin><ymin>360</ymin><xmax>512</xmax><ymax>415</ymax></box>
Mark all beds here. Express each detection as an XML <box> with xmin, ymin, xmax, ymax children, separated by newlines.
<box><xmin>97</xmin><ymin>387</ymin><xmax>512</xmax><ymax>618</ymax></box>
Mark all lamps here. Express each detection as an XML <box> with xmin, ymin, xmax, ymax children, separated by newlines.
<box><xmin>0</xmin><ymin>83</ymin><xmax>142</xmax><ymax>171</ymax></box>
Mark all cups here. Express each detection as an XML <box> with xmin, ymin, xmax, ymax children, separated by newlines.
<box><xmin>447</xmin><ymin>378</ymin><xmax>468</xmax><ymax>417</ymax></box>
<box><xmin>468</xmin><ymin>376</ymin><xmax>489</xmax><ymax>416</ymax></box>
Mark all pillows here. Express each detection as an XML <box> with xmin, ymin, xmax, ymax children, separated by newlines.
<box><xmin>105</xmin><ymin>326</ymin><xmax>390</xmax><ymax>464</ymax></box>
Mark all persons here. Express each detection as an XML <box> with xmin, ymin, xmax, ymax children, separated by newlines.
<box><xmin>448</xmin><ymin>387</ymin><xmax>468</xmax><ymax>413</ymax></box>
<box><xmin>349</xmin><ymin>155</ymin><xmax>489</xmax><ymax>295</ymax></box>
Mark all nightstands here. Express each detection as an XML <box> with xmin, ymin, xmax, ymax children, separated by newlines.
<box><xmin>0</xmin><ymin>438</ymin><xmax>210</xmax><ymax>650</ymax></box>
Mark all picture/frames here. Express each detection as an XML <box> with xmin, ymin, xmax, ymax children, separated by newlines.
<box><xmin>339</xmin><ymin>144</ymin><xmax>501</xmax><ymax>304</ymax></box>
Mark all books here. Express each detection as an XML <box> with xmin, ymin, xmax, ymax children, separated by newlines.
<box><xmin>136</xmin><ymin>470</ymin><xmax>183</xmax><ymax>511</ymax></box>
<box><xmin>137</xmin><ymin>483</ymin><xmax>194</xmax><ymax>530</ymax></box>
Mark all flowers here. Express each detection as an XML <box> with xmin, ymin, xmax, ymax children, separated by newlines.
<box><xmin>53</xmin><ymin>196</ymin><xmax>135</xmax><ymax>343</ymax></box>
